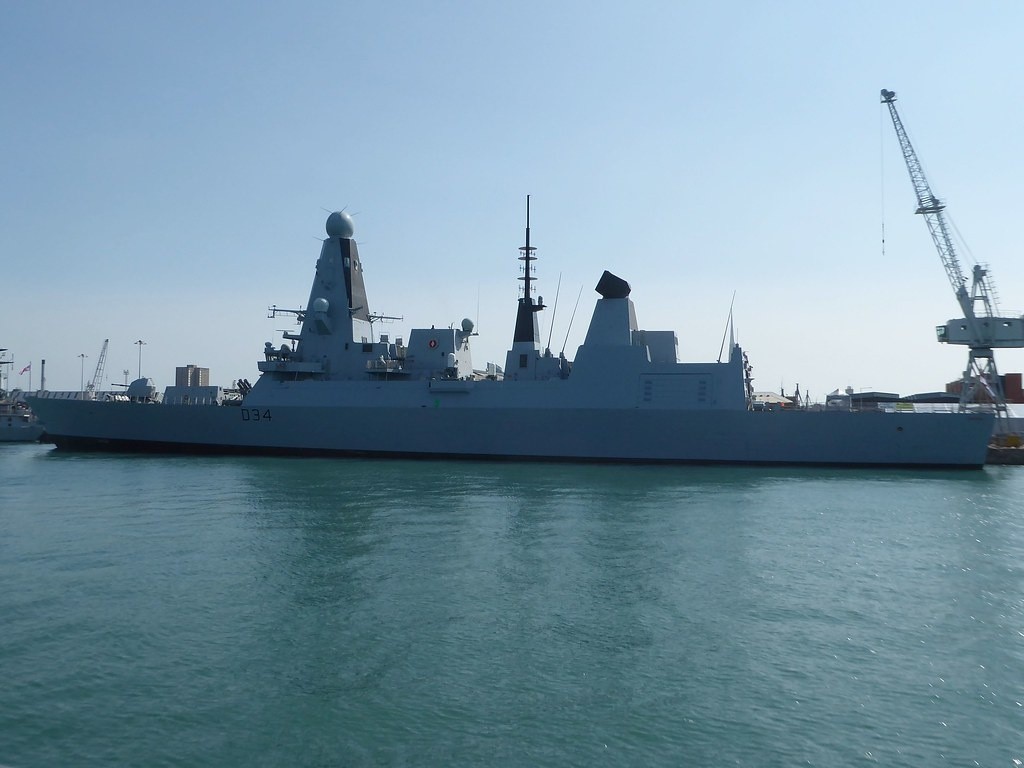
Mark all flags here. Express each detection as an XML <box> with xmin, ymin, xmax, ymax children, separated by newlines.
<box><xmin>19</xmin><ymin>365</ymin><xmax>31</xmax><ymax>375</ymax></box>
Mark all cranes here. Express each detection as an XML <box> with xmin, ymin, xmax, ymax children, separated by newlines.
<box><xmin>874</xmin><ymin>87</ymin><xmax>1024</xmax><ymax>436</ymax></box>
<box><xmin>85</xmin><ymin>338</ymin><xmax>111</xmax><ymax>401</ymax></box>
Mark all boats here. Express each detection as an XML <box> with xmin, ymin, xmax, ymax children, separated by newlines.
<box><xmin>0</xmin><ymin>401</ymin><xmax>46</xmax><ymax>443</ymax></box>
<box><xmin>22</xmin><ymin>191</ymin><xmax>996</xmax><ymax>467</ymax></box>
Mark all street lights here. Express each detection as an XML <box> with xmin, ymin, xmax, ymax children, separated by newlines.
<box><xmin>77</xmin><ymin>353</ymin><xmax>88</xmax><ymax>400</ymax></box>
<box><xmin>134</xmin><ymin>340</ymin><xmax>148</xmax><ymax>379</ymax></box>
